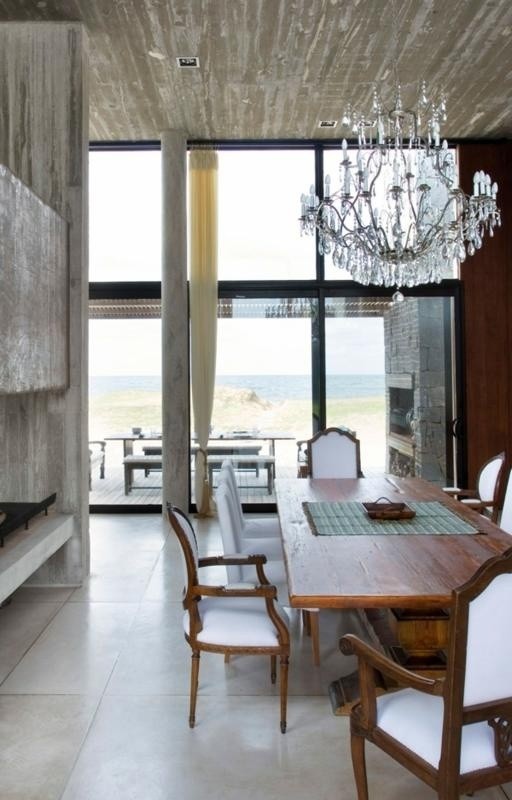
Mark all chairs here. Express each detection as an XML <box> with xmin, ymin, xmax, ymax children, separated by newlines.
<box><xmin>442</xmin><ymin>452</ymin><xmax>511</xmax><ymax>533</ymax></box>
<box><xmin>338</xmin><ymin>547</ymin><xmax>511</xmax><ymax>798</ymax></box>
<box><xmin>166</xmin><ymin>501</ymin><xmax>290</xmax><ymax>733</ymax></box>
<box><xmin>215</xmin><ymin>457</ymin><xmax>289</xmax><ymax>661</ymax></box>
<box><xmin>307</xmin><ymin>428</ymin><xmax>363</xmax><ymax>478</ymax></box>
<box><xmin>89</xmin><ymin>440</ymin><xmax>106</xmax><ymax>495</ymax></box>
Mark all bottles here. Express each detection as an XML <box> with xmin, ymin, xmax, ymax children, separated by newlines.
<box><xmin>406</xmin><ymin>406</ymin><xmax>415</xmax><ymax>440</ymax></box>
<box><xmin>392</xmin><ymin>452</ymin><xmax>415</xmax><ymax>477</ymax></box>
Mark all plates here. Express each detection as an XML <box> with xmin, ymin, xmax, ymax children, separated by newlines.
<box><xmin>362</xmin><ymin>497</ymin><xmax>416</xmax><ymax>519</ymax></box>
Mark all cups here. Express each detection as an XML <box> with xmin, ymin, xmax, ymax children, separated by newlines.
<box><xmin>133</xmin><ymin>428</ymin><xmax>141</xmax><ymax>434</ymax></box>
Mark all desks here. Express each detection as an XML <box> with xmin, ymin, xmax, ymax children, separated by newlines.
<box><xmin>104</xmin><ymin>428</ymin><xmax>298</xmax><ymax>487</ymax></box>
<box><xmin>274</xmin><ymin>477</ymin><xmax>512</xmax><ymax>716</ymax></box>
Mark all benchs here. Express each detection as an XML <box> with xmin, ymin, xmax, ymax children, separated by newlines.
<box><xmin>121</xmin><ymin>452</ymin><xmax>193</xmax><ymax>496</ymax></box>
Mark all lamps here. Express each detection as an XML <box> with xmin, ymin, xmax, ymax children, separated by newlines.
<box><xmin>296</xmin><ymin>59</ymin><xmax>507</xmax><ymax>305</ymax></box>
